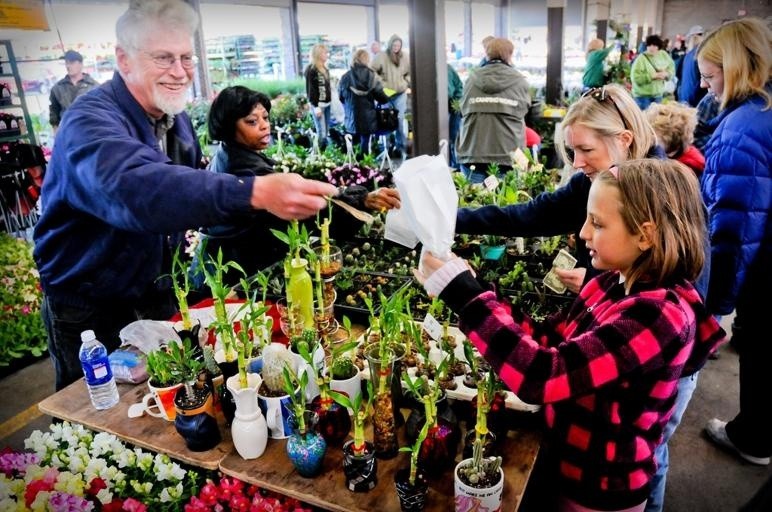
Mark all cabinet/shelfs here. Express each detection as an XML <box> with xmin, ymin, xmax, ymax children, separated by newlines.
<box><xmin>0</xmin><ymin>60</ymin><xmax>30</xmax><ymax>144</ymax></box>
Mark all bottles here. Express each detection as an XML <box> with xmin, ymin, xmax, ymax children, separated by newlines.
<box><xmin>78</xmin><ymin>329</ymin><xmax>119</xmax><ymax>411</ymax></box>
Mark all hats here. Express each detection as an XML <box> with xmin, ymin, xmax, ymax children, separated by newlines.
<box><xmin>59</xmin><ymin>50</ymin><xmax>82</xmax><ymax>62</ymax></box>
<box><xmin>686</xmin><ymin>25</ymin><xmax>704</xmax><ymax>39</ymax></box>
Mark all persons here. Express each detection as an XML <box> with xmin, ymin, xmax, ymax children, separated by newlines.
<box><xmin>448</xmin><ymin>37</ymin><xmax>546</xmax><ymax>179</ymax></box>
<box><xmin>413</xmin><ymin>158</ymin><xmax>727</xmax><ymax>512</ymax></box>
<box><xmin>582</xmin><ymin>30</ymin><xmax>708</xmax><ymax>168</ymax></box>
<box><xmin>694</xmin><ymin>17</ymin><xmax>772</xmax><ymax>467</ymax></box>
<box><xmin>34</xmin><ymin>0</ymin><xmax>340</xmax><ymax>432</ymax></box>
<box><xmin>305</xmin><ymin>32</ymin><xmax>411</xmax><ymax>153</ymax></box>
<box><xmin>457</xmin><ymin>80</ymin><xmax>712</xmax><ymax>512</ymax></box>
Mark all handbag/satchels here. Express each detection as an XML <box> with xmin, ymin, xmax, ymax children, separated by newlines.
<box><xmin>375</xmin><ymin>101</ymin><xmax>399</xmax><ymax>135</ymax></box>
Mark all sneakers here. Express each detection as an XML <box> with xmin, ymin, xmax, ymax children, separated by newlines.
<box><xmin>705</xmin><ymin>418</ymin><xmax>770</xmax><ymax>464</ymax></box>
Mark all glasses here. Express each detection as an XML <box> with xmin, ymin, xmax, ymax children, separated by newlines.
<box><xmin>580</xmin><ymin>88</ymin><xmax>633</xmax><ymax>155</ymax></box>
<box><xmin>141</xmin><ymin>50</ymin><xmax>199</xmax><ymax>69</ymax></box>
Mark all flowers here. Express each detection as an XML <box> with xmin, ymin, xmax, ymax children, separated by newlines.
<box><xmin>0</xmin><ymin>232</ymin><xmax>44</xmax><ymax>363</ymax></box>
<box><xmin>0</xmin><ymin>415</ymin><xmax>145</xmax><ymax>511</ymax></box>
<box><xmin>280</xmin><ymin>133</ymin><xmax>394</xmax><ymax>188</ymax></box>
<box><xmin>451</xmin><ymin>145</ymin><xmax>554</xmax><ymax>205</ymax></box>
